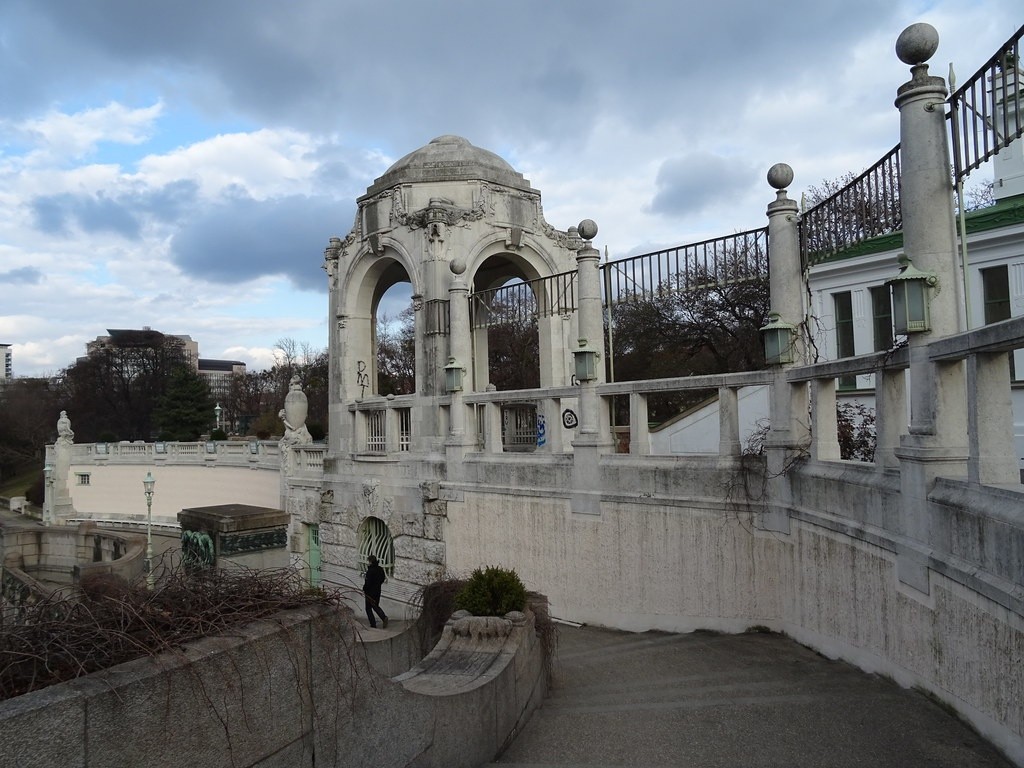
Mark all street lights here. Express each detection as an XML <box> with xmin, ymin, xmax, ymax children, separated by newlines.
<box><xmin>214</xmin><ymin>402</ymin><xmax>222</xmax><ymax>430</ymax></box>
<box><xmin>142</xmin><ymin>471</ymin><xmax>157</xmax><ymax>590</ymax></box>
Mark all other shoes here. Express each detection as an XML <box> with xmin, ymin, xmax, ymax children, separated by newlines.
<box><xmin>383</xmin><ymin>616</ymin><xmax>389</xmax><ymax>629</ymax></box>
<box><xmin>370</xmin><ymin>624</ymin><xmax>377</xmax><ymax>628</ymax></box>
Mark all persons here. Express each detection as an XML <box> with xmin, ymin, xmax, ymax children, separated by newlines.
<box><xmin>363</xmin><ymin>555</ymin><xmax>389</xmax><ymax>629</ymax></box>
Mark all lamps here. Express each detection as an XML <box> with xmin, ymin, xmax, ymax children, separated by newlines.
<box><xmin>572</xmin><ymin>337</ymin><xmax>599</xmax><ymax>381</ymax></box>
<box><xmin>246</xmin><ymin>441</ymin><xmax>259</xmax><ymax>456</ymax></box>
<box><xmin>96</xmin><ymin>443</ymin><xmax>105</xmax><ymax>454</ymax></box>
<box><xmin>205</xmin><ymin>441</ymin><xmax>216</xmax><ymax>454</ymax></box>
<box><xmin>760</xmin><ymin>310</ymin><xmax>794</xmax><ymax>364</ymax></box>
<box><xmin>155</xmin><ymin>442</ymin><xmax>165</xmax><ymax>454</ymax></box>
<box><xmin>886</xmin><ymin>253</ymin><xmax>936</xmax><ymax>336</ymax></box>
<box><xmin>444</xmin><ymin>356</ymin><xmax>465</xmax><ymax>392</ymax></box>
